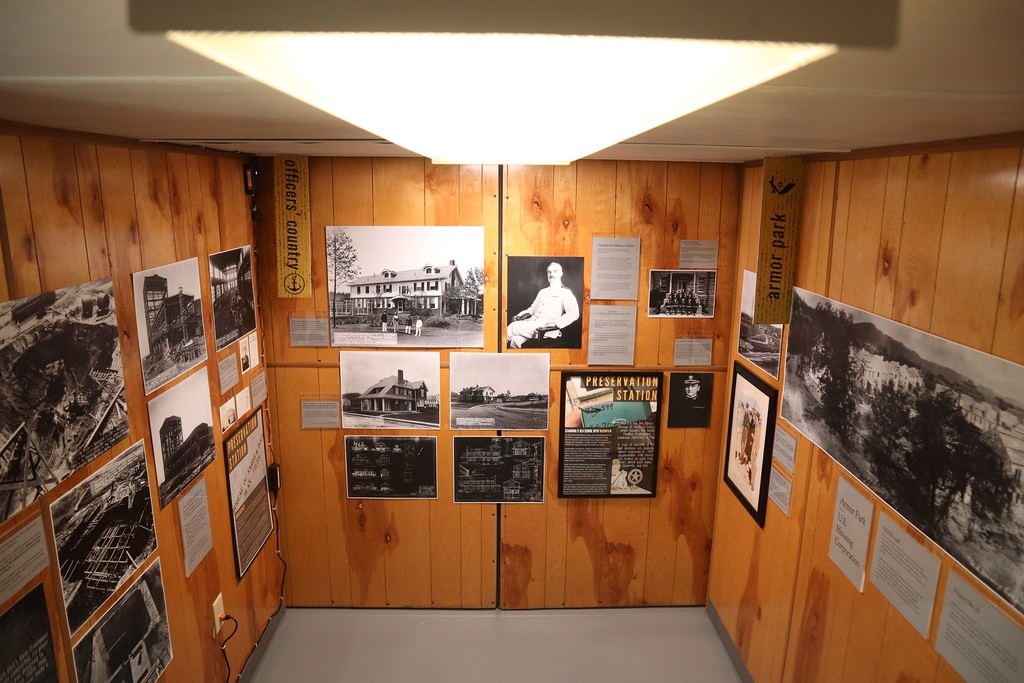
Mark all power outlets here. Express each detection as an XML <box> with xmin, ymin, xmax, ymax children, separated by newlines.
<box><xmin>213</xmin><ymin>591</ymin><xmax>227</xmax><ymax>635</ymax></box>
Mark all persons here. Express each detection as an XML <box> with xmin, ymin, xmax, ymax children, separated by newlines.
<box><xmin>665</xmin><ymin>289</ymin><xmax>699</xmax><ymax>316</ymax></box>
<box><xmin>405</xmin><ymin>315</ymin><xmax>412</xmax><ymax>335</ymax></box>
<box><xmin>381</xmin><ymin>309</ymin><xmax>388</xmax><ymax>333</ymax></box>
<box><xmin>675</xmin><ymin>375</ymin><xmax>707</xmax><ymax>427</ymax></box>
<box><xmin>507</xmin><ymin>260</ymin><xmax>579</xmax><ymax>350</ymax></box>
<box><xmin>415</xmin><ymin>318</ymin><xmax>423</xmax><ymax>337</ymax></box>
<box><xmin>392</xmin><ymin>312</ymin><xmax>398</xmax><ymax>332</ymax></box>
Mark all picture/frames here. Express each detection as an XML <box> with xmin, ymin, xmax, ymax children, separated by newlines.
<box><xmin>721</xmin><ymin>360</ymin><xmax>780</xmax><ymax>528</ymax></box>
